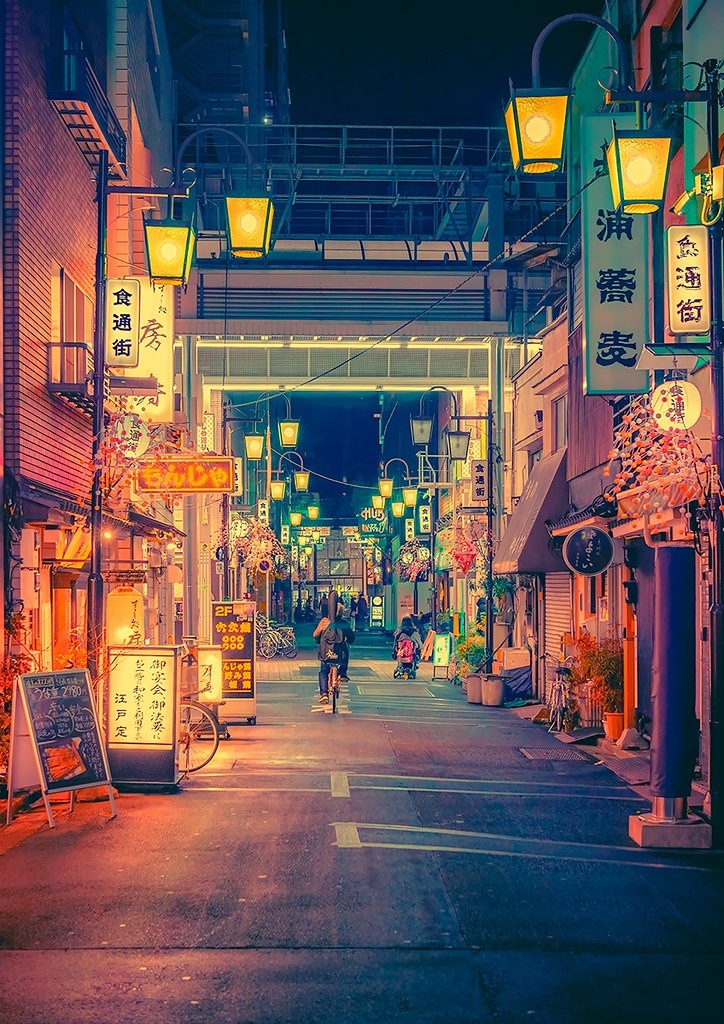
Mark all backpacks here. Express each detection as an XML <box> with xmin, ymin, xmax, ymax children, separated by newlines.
<box><xmin>397</xmin><ymin>630</ymin><xmax>414</xmax><ymax>664</ymax></box>
<box><xmin>318</xmin><ymin>630</ymin><xmax>342</xmax><ymax>663</ymax></box>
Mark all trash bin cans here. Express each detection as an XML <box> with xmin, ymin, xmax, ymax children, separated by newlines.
<box><xmin>480</xmin><ymin>674</ymin><xmax>503</xmax><ymax>706</ymax></box>
<box><xmin>464</xmin><ymin>673</ymin><xmax>481</xmax><ymax>703</ymax></box>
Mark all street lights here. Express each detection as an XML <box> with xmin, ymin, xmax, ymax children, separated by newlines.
<box><xmin>379</xmin><ymin>457</ymin><xmax>418</xmax><ymax>613</ymax></box>
<box><xmin>500</xmin><ymin>11</ymin><xmax>724</xmax><ymax>851</ymax></box>
<box><xmin>409</xmin><ymin>385</ymin><xmax>495</xmax><ymax>676</ymax></box>
<box><xmin>256</xmin><ymin>450</ymin><xmax>311</xmax><ymax>609</ymax></box>
<box><xmin>83</xmin><ymin>127</ymin><xmax>270</xmax><ymax>686</ymax></box>
<box><xmin>400</xmin><ymin>442</ymin><xmax>436</xmax><ymax>635</ymax></box>
<box><xmin>223</xmin><ymin>389</ymin><xmax>301</xmax><ymax>603</ymax></box>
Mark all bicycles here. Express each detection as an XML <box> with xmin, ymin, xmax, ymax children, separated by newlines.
<box><xmin>255</xmin><ymin>609</ymin><xmax>317</xmax><ymax>662</ymax></box>
<box><xmin>178</xmin><ymin>691</ymin><xmax>221</xmax><ymax>780</ymax></box>
<box><xmin>318</xmin><ymin>662</ymin><xmax>350</xmax><ymax>714</ymax></box>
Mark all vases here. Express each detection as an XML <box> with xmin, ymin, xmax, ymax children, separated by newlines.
<box><xmin>588</xmin><ymin>646</ymin><xmax>624</xmax><ymax>739</ymax></box>
<box><xmin>465</xmin><ymin>673</ymin><xmax>504</xmax><ymax>706</ymax></box>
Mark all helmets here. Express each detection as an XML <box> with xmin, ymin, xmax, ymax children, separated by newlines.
<box><xmin>401</xmin><ymin>617</ymin><xmax>415</xmax><ymax>627</ymax></box>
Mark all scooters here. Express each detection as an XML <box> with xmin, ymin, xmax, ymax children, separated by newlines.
<box><xmin>394</xmin><ymin>637</ymin><xmax>419</xmax><ymax>677</ymax></box>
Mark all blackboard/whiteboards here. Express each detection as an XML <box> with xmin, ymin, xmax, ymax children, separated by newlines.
<box><xmin>9</xmin><ymin>668</ymin><xmax>111</xmax><ymax>794</ymax></box>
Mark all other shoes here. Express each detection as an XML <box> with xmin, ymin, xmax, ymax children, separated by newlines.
<box><xmin>318</xmin><ymin>695</ymin><xmax>327</xmax><ymax>703</ymax></box>
<box><xmin>340</xmin><ymin>676</ymin><xmax>350</xmax><ymax>681</ymax></box>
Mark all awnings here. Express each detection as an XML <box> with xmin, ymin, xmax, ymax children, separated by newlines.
<box><xmin>492</xmin><ymin>447</ymin><xmax>571</xmax><ymax>574</ymax></box>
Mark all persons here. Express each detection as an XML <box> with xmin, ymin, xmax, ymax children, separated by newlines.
<box><xmin>306</xmin><ymin>596</ymin><xmax>312</xmax><ymax>606</ymax></box>
<box><xmin>313</xmin><ymin>603</ymin><xmax>354</xmax><ymax>701</ymax></box>
<box><xmin>394</xmin><ymin>617</ymin><xmax>423</xmax><ymax>668</ymax></box>
<box><xmin>338</xmin><ymin>595</ymin><xmax>344</xmax><ymax>604</ymax></box>
<box><xmin>318</xmin><ymin>594</ymin><xmax>328</xmax><ymax>618</ymax></box>
<box><xmin>351</xmin><ymin>593</ymin><xmax>367</xmax><ymax>620</ymax></box>
